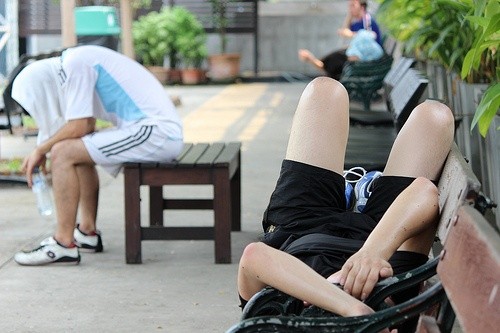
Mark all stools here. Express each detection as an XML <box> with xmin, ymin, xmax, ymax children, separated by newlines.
<box><xmin>125</xmin><ymin>142</ymin><xmax>242</xmax><ymax>263</ymax></box>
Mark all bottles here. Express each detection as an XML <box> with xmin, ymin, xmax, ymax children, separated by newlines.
<box><xmin>31</xmin><ymin>166</ymin><xmax>53</xmax><ymax>216</ymax></box>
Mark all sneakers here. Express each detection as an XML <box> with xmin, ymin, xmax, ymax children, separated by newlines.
<box><xmin>343</xmin><ymin>166</ymin><xmax>367</xmax><ymax>210</ymax></box>
<box><xmin>353</xmin><ymin>170</ymin><xmax>383</xmax><ymax>214</ymax></box>
<box><xmin>15</xmin><ymin>236</ymin><xmax>81</xmax><ymax>267</ymax></box>
<box><xmin>73</xmin><ymin>223</ymin><xmax>104</xmax><ymax>253</ymax></box>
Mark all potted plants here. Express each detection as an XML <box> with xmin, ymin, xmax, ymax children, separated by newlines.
<box><xmin>206</xmin><ymin>2</ymin><xmax>241</xmax><ymax>82</ymax></box>
<box><xmin>133</xmin><ymin>6</ymin><xmax>206</xmax><ymax>85</ymax></box>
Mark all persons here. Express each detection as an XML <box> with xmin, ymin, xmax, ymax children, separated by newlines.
<box><xmin>3</xmin><ymin>45</ymin><xmax>183</xmax><ymax>266</ymax></box>
<box><xmin>298</xmin><ymin>0</ymin><xmax>385</xmax><ymax>82</ymax></box>
<box><xmin>238</xmin><ymin>76</ymin><xmax>455</xmax><ymax>333</ymax></box>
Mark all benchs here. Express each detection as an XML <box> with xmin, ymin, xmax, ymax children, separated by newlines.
<box><xmin>225</xmin><ymin>137</ymin><xmax>500</xmax><ymax>333</ymax></box>
<box><xmin>333</xmin><ymin>29</ymin><xmax>429</xmax><ymax>170</ymax></box>
